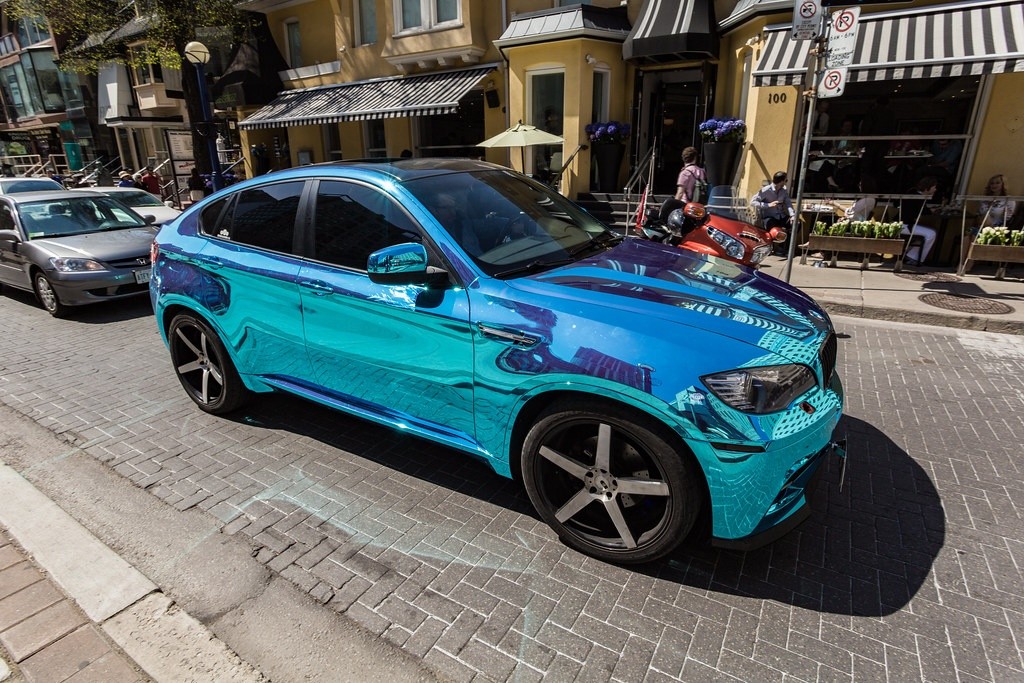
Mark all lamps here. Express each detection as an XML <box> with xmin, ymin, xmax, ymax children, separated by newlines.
<box><xmin>586</xmin><ymin>53</ymin><xmax>597</xmax><ymax>65</ymax></box>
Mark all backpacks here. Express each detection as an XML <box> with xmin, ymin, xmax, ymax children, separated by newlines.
<box><xmin>681</xmin><ymin>168</ymin><xmax>709</xmax><ymax>205</ymax></box>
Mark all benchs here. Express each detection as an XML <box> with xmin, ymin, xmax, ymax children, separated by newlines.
<box><xmin>35</xmin><ymin>215</ymin><xmax>80</xmax><ymax>235</ymax></box>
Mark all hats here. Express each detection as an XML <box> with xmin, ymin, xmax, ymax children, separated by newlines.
<box><xmin>118</xmin><ymin>170</ymin><xmax>130</xmax><ymax>179</ymax></box>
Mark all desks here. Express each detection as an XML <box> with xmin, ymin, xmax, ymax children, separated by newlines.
<box><xmin>920</xmin><ymin>206</ymin><xmax>978</xmax><ymax>268</ymax></box>
<box><xmin>798</xmin><ymin>210</ymin><xmax>837</xmax><ymax>235</ymax></box>
<box><xmin>883</xmin><ymin>153</ymin><xmax>934</xmax><ymax>194</ymax></box>
<box><xmin>815</xmin><ymin>154</ymin><xmax>858</xmax><ymax>192</ymax></box>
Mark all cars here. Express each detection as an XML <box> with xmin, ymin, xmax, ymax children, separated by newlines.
<box><xmin>0</xmin><ymin>177</ymin><xmax>185</xmax><ymax>318</ymax></box>
<box><xmin>150</xmin><ymin>157</ymin><xmax>849</xmax><ymax>562</ymax></box>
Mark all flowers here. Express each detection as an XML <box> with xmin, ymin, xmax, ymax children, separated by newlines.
<box><xmin>976</xmin><ymin>226</ymin><xmax>1024</xmax><ymax>247</ymax></box>
<box><xmin>583</xmin><ymin>121</ymin><xmax>632</xmax><ymax>144</ymax></box>
<box><xmin>698</xmin><ymin>116</ymin><xmax>745</xmax><ymax>144</ymax></box>
<box><xmin>812</xmin><ymin>219</ymin><xmax>909</xmax><ymax>239</ymax></box>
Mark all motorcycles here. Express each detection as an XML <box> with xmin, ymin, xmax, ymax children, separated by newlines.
<box><xmin>639</xmin><ymin>187</ymin><xmax>787</xmax><ymax>273</ymax></box>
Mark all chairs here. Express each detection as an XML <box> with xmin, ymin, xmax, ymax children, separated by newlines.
<box><xmin>537</xmin><ymin>152</ymin><xmax>563</xmax><ymax>189</ymax></box>
<box><xmin>20</xmin><ymin>215</ymin><xmax>41</xmax><ymax>236</ymax></box>
<box><xmin>902</xmin><ymin>233</ymin><xmax>925</xmax><ymax>266</ymax></box>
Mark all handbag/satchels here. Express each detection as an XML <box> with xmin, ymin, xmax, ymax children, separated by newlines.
<box><xmin>189</xmin><ymin>189</ymin><xmax>205</xmax><ymax>202</ymax></box>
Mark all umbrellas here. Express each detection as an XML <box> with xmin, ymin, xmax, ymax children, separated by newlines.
<box><xmin>475</xmin><ymin>120</ymin><xmax>566</xmax><ymax>174</ymax></box>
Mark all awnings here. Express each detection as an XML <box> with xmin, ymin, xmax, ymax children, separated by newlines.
<box><xmin>237</xmin><ymin>61</ymin><xmax>498</xmax><ymax>131</ymax></box>
<box><xmin>752</xmin><ymin>0</ymin><xmax>1024</xmax><ymax>87</ymax></box>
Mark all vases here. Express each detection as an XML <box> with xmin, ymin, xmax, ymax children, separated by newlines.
<box><xmin>809</xmin><ymin>233</ymin><xmax>905</xmax><ymax>255</ymax></box>
<box><xmin>703</xmin><ymin>141</ymin><xmax>739</xmax><ymax>188</ymax></box>
<box><xmin>969</xmin><ymin>242</ymin><xmax>1024</xmax><ymax>263</ymax></box>
<box><xmin>595</xmin><ymin>144</ymin><xmax>626</xmax><ymax>192</ymax></box>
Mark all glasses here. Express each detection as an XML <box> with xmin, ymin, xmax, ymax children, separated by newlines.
<box><xmin>137</xmin><ymin>177</ymin><xmax>141</xmax><ymax>179</ymax></box>
<box><xmin>785</xmin><ymin>179</ymin><xmax>789</xmax><ymax>183</ymax></box>
<box><xmin>125</xmin><ymin>176</ymin><xmax>128</xmax><ymax>177</ymax></box>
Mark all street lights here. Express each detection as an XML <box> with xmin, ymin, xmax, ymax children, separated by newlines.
<box><xmin>185</xmin><ymin>39</ymin><xmax>224</xmax><ymax>193</ymax></box>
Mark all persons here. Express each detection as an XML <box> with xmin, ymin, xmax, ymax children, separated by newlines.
<box><xmin>400</xmin><ymin>150</ymin><xmax>413</xmax><ymax>158</ymax></box>
<box><xmin>901</xmin><ymin>175</ymin><xmax>936</xmax><ymax>267</ymax></box>
<box><xmin>831</xmin><ymin>118</ymin><xmax>860</xmax><ymax>181</ymax></box>
<box><xmin>422</xmin><ymin>191</ymin><xmax>485</xmax><ymax>286</ymax></box>
<box><xmin>132</xmin><ymin>173</ymin><xmax>150</xmax><ymax>191</ymax></box>
<box><xmin>188</xmin><ymin>168</ymin><xmax>206</xmax><ymax>204</ymax></box>
<box><xmin>674</xmin><ymin>147</ymin><xmax>708</xmax><ymax>203</ymax></box>
<box><xmin>95</xmin><ymin>161</ymin><xmax>114</xmax><ymax>186</ymax></box>
<box><xmin>142</xmin><ymin>166</ymin><xmax>162</xmax><ymax>201</ymax></box>
<box><xmin>925</xmin><ymin>130</ymin><xmax>961</xmax><ymax>197</ymax></box>
<box><xmin>882</xmin><ymin>124</ymin><xmax>919</xmax><ymax>194</ymax></box>
<box><xmin>750</xmin><ymin>170</ymin><xmax>801</xmax><ymax>255</ymax></box>
<box><xmin>954</xmin><ymin>175</ymin><xmax>1016</xmax><ymax>273</ymax></box>
<box><xmin>118</xmin><ymin>171</ymin><xmax>136</xmax><ymax>187</ymax></box>
<box><xmin>798</xmin><ymin>125</ymin><xmax>841</xmax><ymax>188</ymax></box>
<box><xmin>801</xmin><ymin>99</ymin><xmax>829</xmax><ymax>147</ymax></box>
<box><xmin>78</xmin><ymin>205</ymin><xmax>108</xmax><ymax>230</ymax></box>
<box><xmin>48</xmin><ymin>170</ymin><xmax>63</xmax><ymax>184</ymax></box>
